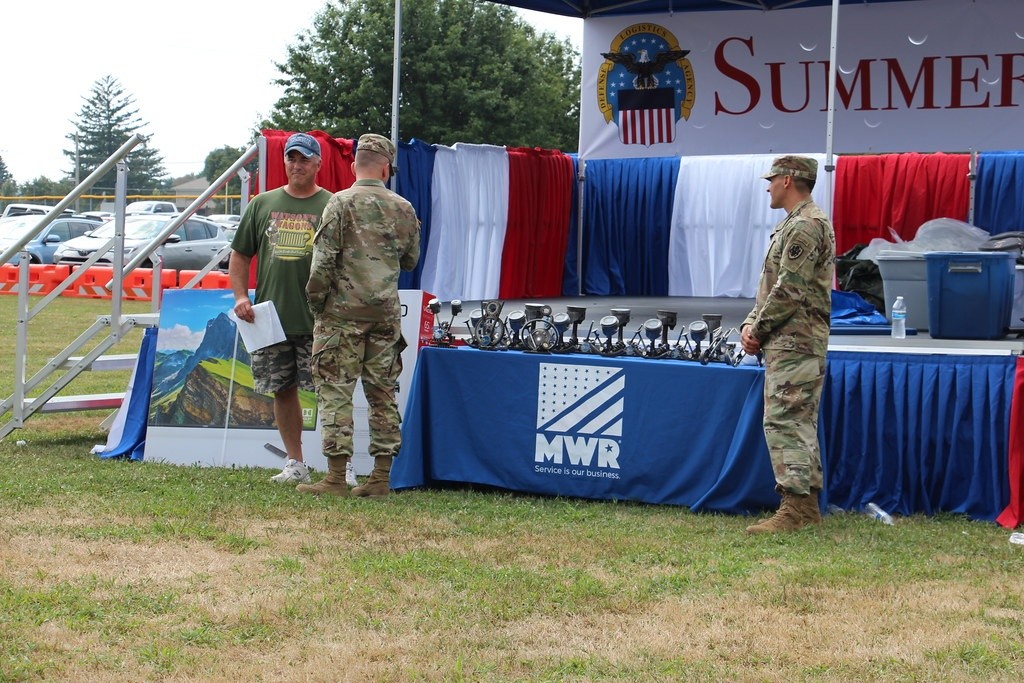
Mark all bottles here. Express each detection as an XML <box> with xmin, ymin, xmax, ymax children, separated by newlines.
<box><xmin>891</xmin><ymin>296</ymin><xmax>906</xmax><ymax>338</ymax></box>
<box><xmin>865</xmin><ymin>503</ymin><xmax>895</xmax><ymax>528</ymax></box>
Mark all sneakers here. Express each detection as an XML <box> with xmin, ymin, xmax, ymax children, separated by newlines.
<box><xmin>345</xmin><ymin>462</ymin><xmax>359</xmax><ymax>486</ymax></box>
<box><xmin>269</xmin><ymin>457</ymin><xmax>312</xmax><ymax>485</ymax></box>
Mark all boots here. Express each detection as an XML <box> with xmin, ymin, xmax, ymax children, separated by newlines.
<box><xmin>295</xmin><ymin>456</ymin><xmax>352</xmax><ymax>497</ymax></box>
<box><xmin>745</xmin><ymin>491</ymin><xmax>803</xmax><ymax>535</ymax></box>
<box><xmin>757</xmin><ymin>488</ymin><xmax>822</xmax><ymax>527</ymax></box>
<box><xmin>350</xmin><ymin>455</ymin><xmax>392</xmax><ymax>497</ymax></box>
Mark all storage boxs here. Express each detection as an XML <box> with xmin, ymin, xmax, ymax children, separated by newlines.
<box><xmin>880</xmin><ymin>249</ymin><xmax>1024</xmax><ymax>339</ymax></box>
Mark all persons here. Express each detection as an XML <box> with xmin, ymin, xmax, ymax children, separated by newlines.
<box><xmin>296</xmin><ymin>134</ymin><xmax>421</xmax><ymax>497</ymax></box>
<box><xmin>228</xmin><ymin>134</ymin><xmax>356</xmax><ymax>485</ymax></box>
<box><xmin>741</xmin><ymin>156</ymin><xmax>835</xmax><ymax>534</ymax></box>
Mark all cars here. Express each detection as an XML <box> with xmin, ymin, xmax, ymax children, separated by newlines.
<box><xmin>1</xmin><ymin>200</ymin><xmax>243</xmax><ymax>271</ymax></box>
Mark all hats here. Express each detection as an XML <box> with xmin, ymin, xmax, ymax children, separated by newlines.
<box><xmin>760</xmin><ymin>155</ymin><xmax>818</xmax><ymax>181</ymax></box>
<box><xmin>284</xmin><ymin>133</ymin><xmax>321</xmax><ymax>159</ymax></box>
<box><xmin>356</xmin><ymin>133</ymin><xmax>397</xmax><ymax>176</ymax></box>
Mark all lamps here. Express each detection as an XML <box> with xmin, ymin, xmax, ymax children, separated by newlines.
<box><xmin>423</xmin><ymin>298</ymin><xmax>765</xmax><ymax>368</ymax></box>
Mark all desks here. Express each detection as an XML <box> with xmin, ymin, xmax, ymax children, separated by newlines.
<box><xmin>386</xmin><ymin>342</ymin><xmax>826</xmax><ymax>519</ymax></box>
